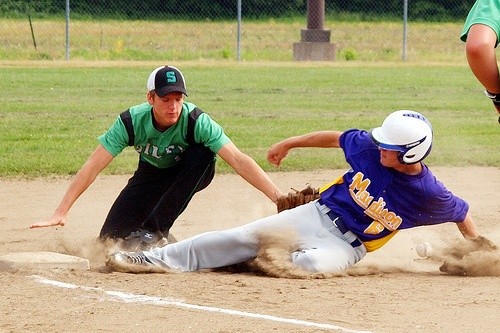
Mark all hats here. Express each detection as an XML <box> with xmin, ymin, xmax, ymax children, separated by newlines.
<box><xmin>147</xmin><ymin>65</ymin><xmax>188</xmax><ymax>98</ymax></box>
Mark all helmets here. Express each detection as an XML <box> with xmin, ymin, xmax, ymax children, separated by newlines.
<box><xmin>370</xmin><ymin>110</ymin><xmax>433</xmax><ymax>165</ymax></box>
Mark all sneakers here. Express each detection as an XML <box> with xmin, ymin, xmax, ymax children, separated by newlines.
<box><xmin>126</xmin><ymin>230</ymin><xmax>157</xmax><ymax>251</ymax></box>
<box><xmin>115</xmin><ymin>251</ymin><xmax>152</xmax><ymax>266</ymax></box>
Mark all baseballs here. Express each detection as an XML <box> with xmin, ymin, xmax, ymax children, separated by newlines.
<box><xmin>416</xmin><ymin>242</ymin><xmax>432</xmax><ymax>257</ymax></box>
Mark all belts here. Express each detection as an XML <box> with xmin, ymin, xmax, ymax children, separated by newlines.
<box><xmin>317</xmin><ymin>198</ymin><xmax>362</xmax><ymax>248</ymax></box>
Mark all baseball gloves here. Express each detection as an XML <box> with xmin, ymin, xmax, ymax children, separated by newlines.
<box><xmin>277</xmin><ymin>187</ymin><xmax>320</xmax><ymax>213</ymax></box>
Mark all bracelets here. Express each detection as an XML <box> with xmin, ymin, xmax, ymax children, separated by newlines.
<box><xmin>485</xmin><ymin>90</ymin><xmax>500</xmax><ymax>111</ymax></box>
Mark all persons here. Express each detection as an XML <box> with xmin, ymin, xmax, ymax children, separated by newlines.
<box><xmin>114</xmin><ymin>110</ymin><xmax>479</xmax><ymax>277</ymax></box>
<box><xmin>459</xmin><ymin>0</ymin><xmax>500</xmax><ymax>123</ymax></box>
<box><xmin>30</xmin><ymin>65</ymin><xmax>290</xmax><ymax>251</ymax></box>
